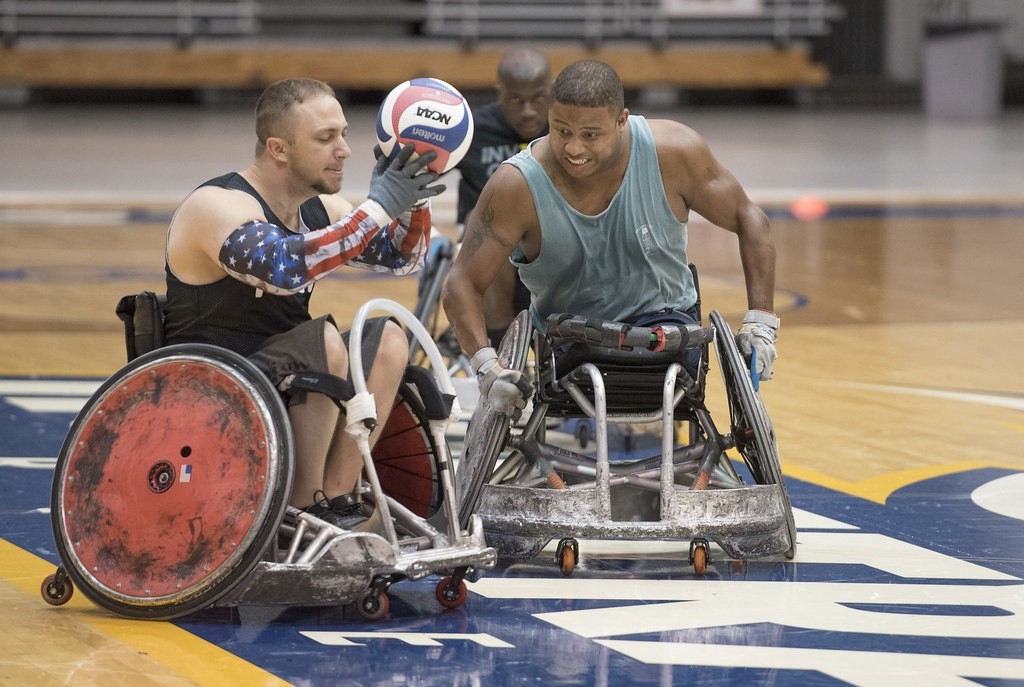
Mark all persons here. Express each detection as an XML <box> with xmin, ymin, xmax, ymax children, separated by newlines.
<box><xmin>164</xmin><ymin>78</ymin><xmax>446</xmax><ymax>538</ymax></box>
<box><xmin>440</xmin><ymin>48</ymin><xmax>781</xmax><ymax>418</ymax></box>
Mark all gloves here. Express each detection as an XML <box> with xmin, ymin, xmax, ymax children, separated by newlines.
<box><xmin>367</xmin><ymin>142</ymin><xmax>446</xmax><ymax>221</ymax></box>
<box><xmin>468</xmin><ymin>347</ymin><xmax>534</xmax><ymax>422</ymax></box>
<box><xmin>736</xmin><ymin>309</ymin><xmax>780</xmax><ymax>392</ymax></box>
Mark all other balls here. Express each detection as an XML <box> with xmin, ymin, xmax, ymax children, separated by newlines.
<box><xmin>374</xmin><ymin>76</ymin><xmax>475</xmax><ymax>175</ymax></box>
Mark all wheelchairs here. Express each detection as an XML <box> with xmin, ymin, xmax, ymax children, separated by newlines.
<box><xmin>453</xmin><ymin>262</ymin><xmax>799</xmax><ymax>581</ymax></box>
<box><xmin>36</xmin><ymin>288</ymin><xmax>499</xmax><ymax>632</ymax></box>
<box><xmin>404</xmin><ymin>235</ymin><xmax>556</xmax><ymax>433</ymax></box>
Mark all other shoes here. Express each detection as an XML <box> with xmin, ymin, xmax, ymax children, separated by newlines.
<box><xmin>298</xmin><ymin>491</ymin><xmax>411</xmax><ymax>548</ymax></box>
